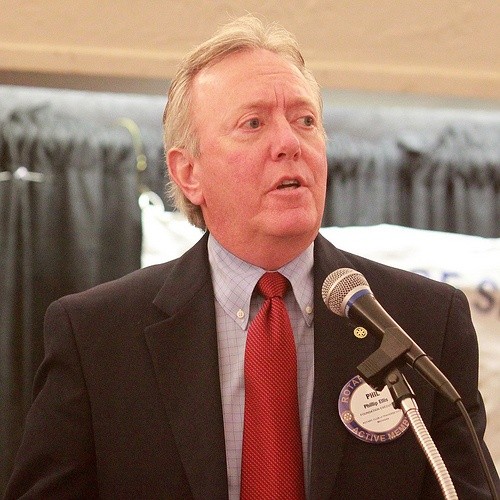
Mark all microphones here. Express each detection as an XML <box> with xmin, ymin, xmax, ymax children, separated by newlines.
<box><xmin>322</xmin><ymin>268</ymin><xmax>461</xmax><ymax>405</ymax></box>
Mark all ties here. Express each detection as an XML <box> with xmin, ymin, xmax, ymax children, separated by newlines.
<box><xmin>238</xmin><ymin>272</ymin><xmax>311</xmax><ymax>499</ymax></box>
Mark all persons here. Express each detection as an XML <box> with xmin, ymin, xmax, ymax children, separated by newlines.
<box><xmin>0</xmin><ymin>17</ymin><xmax>500</xmax><ymax>500</ymax></box>
